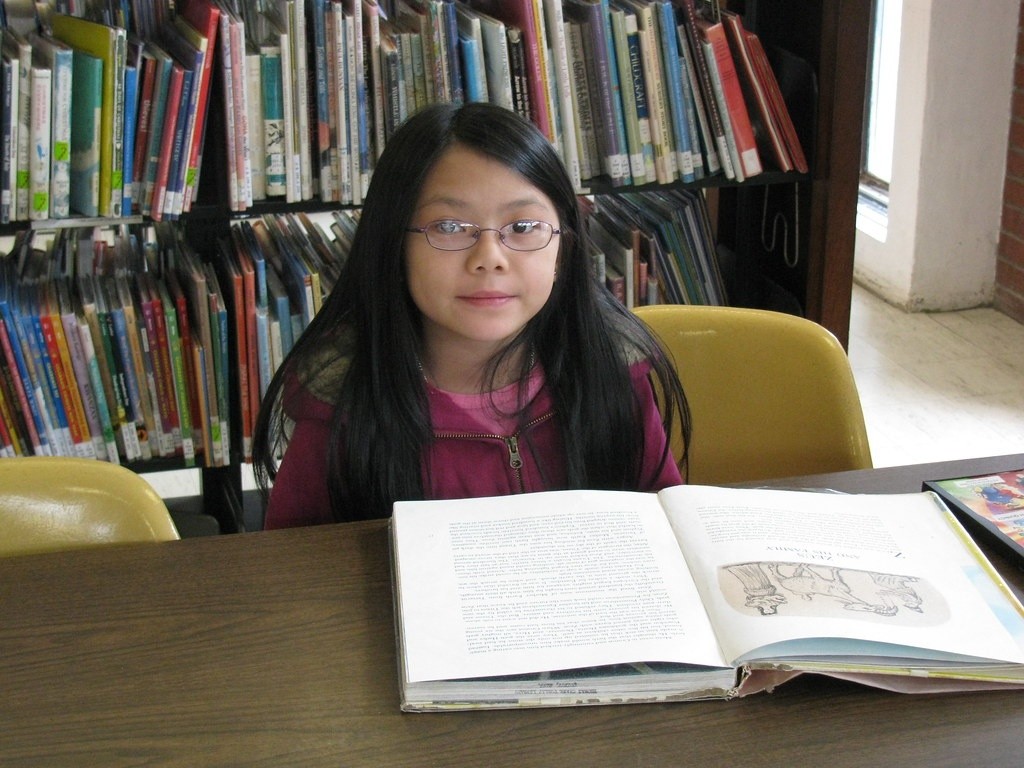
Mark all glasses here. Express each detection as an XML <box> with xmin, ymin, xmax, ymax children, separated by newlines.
<box><xmin>403</xmin><ymin>216</ymin><xmax>563</xmax><ymax>251</ymax></box>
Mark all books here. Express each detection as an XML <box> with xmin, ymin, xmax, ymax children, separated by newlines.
<box><xmin>0</xmin><ymin>0</ymin><xmax>807</xmax><ymax>467</ymax></box>
<box><xmin>388</xmin><ymin>485</ymin><xmax>1024</xmax><ymax>710</ymax></box>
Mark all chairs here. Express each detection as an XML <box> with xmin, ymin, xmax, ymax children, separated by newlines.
<box><xmin>0</xmin><ymin>455</ymin><xmax>178</xmax><ymax>561</ymax></box>
<box><xmin>626</xmin><ymin>305</ymin><xmax>873</xmax><ymax>484</ymax></box>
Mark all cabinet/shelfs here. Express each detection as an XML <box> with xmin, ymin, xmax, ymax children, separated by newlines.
<box><xmin>1</xmin><ymin>1</ymin><xmax>884</xmax><ymax>535</ymax></box>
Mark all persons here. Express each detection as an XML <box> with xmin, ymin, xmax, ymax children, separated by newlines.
<box><xmin>250</xmin><ymin>100</ymin><xmax>688</xmax><ymax>529</ymax></box>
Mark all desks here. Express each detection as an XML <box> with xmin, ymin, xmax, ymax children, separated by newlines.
<box><xmin>0</xmin><ymin>454</ymin><xmax>1023</xmax><ymax>768</ymax></box>
